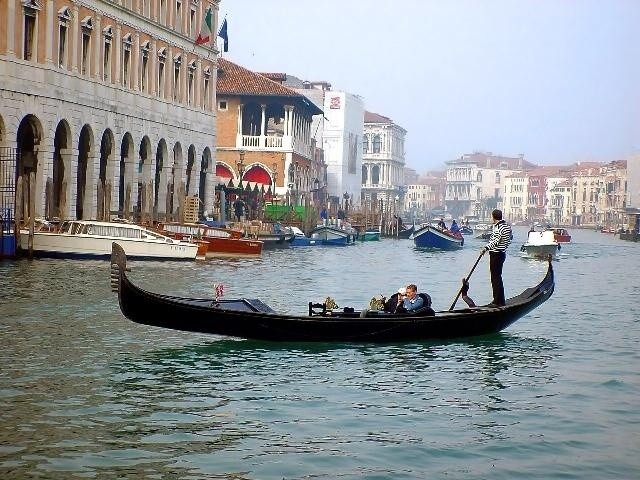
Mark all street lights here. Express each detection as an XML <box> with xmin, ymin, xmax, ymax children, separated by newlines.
<box><xmin>295</xmin><ymin>175</ymin><xmax>299</xmax><ymax>203</ymax></box>
<box><xmin>270</xmin><ymin>163</ymin><xmax>278</xmax><ymax>198</ymax></box>
<box><xmin>236</xmin><ymin>149</ymin><xmax>245</xmax><ymax>212</ymax></box>
<box><xmin>344</xmin><ymin>192</ymin><xmax>350</xmax><ymax>213</ymax></box>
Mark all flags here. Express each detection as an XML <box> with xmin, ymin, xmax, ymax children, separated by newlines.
<box><xmin>194</xmin><ymin>8</ymin><xmax>212</xmax><ymax>45</ymax></box>
<box><xmin>219</xmin><ymin>19</ymin><xmax>229</xmax><ymax>52</ymax></box>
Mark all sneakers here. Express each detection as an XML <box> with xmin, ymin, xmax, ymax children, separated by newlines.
<box><xmin>489</xmin><ymin>303</ymin><xmax>502</xmax><ymax>308</ymax></box>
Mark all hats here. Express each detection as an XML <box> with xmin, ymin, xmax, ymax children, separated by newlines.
<box><xmin>398</xmin><ymin>287</ymin><xmax>407</xmax><ymax>295</ymax></box>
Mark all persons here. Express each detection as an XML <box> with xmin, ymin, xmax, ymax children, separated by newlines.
<box><xmin>465</xmin><ymin>218</ymin><xmax>471</xmax><ymax>228</ymax></box>
<box><xmin>398</xmin><ymin>284</ymin><xmax>424</xmax><ymax>315</ymax></box>
<box><xmin>438</xmin><ymin>218</ymin><xmax>446</xmax><ymax>229</ymax></box>
<box><xmin>233</xmin><ymin>198</ymin><xmax>244</xmax><ymax>222</ymax></box>
<box><xmin>528</xmin><ymin>226</ymin><xmax>536</xmax><ymax>238</ymax></box>
<box><xmin>338</xmin><ymin>206</ymin><xmax>345</xmax><ymax>229</ymax></box>
<box><xmin>450</xmin><ymin>219</ymin><xmax>460</xmax><ymax>234</ymax></box>
<box><xmin>460</xmin><ymin>218</ymin><xmax>464</xmax><ymax>228</ymax></box>
<box><xmin>320</xmin><ymin>205</ymin><xmax>327</xmax><ymax>227</ymax></box>
<box><xmin>482</xmin><ymin>209</ymin><xmax>512</xmax><ymax>308</ymax></box>
<box><xmin>360</xmin><ymin>286</ymin><xmax>407</xmax><ymax>317</ymax></box>
